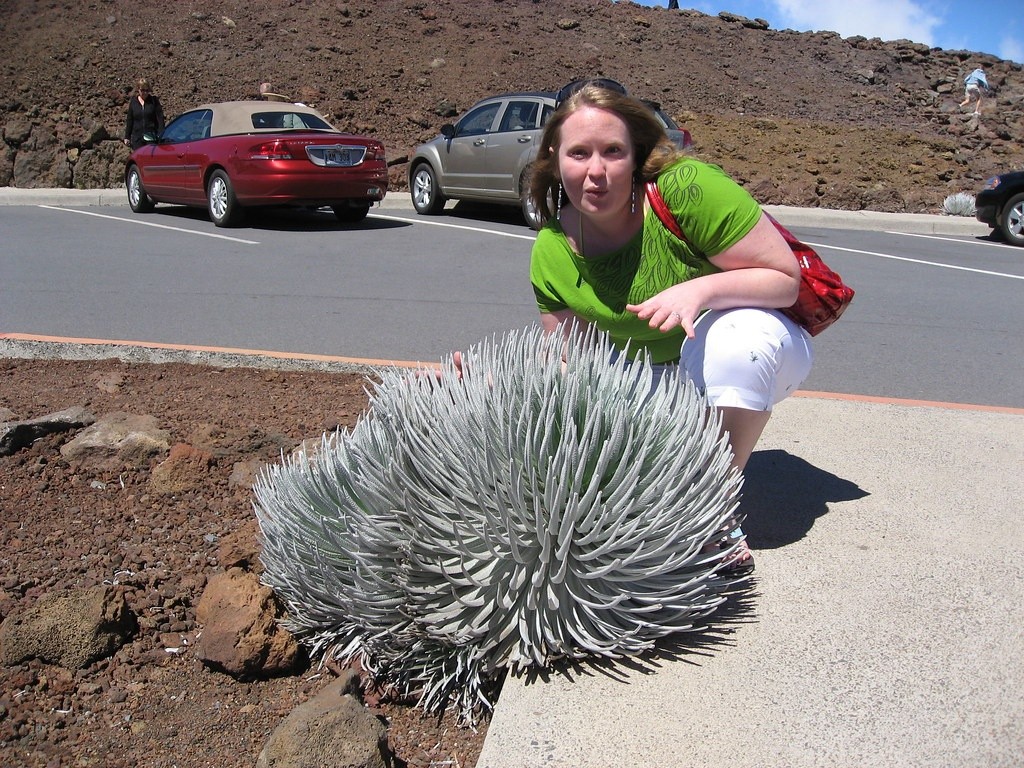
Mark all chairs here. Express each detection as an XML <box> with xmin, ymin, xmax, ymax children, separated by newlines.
<box><xmin>519</xmin><ymin>104</ymin><xmax>546</xmax><ymax>129</ymax></box>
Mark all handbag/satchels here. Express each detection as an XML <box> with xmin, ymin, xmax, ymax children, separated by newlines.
<box><xmin>642</xmin><ymin>170</ymin><xmax>855</xmax><ymax>336</ymax></box>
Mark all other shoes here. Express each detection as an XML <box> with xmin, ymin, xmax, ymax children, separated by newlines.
<box><xmin>956</xmin><ymin>104</ymin><xmax>962</xmax><ymax>109</ymax></box>
<box><xmin>974</xmin><ymin>111</ymin><xmax>982</xmax><ymax>116</ymax></box>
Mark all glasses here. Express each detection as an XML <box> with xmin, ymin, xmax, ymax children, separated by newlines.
<box><xmin>554</xmin><ymin>78</ymin><xmax>626</xmax><ymax>110</ymax></box>
<box><xmin>139</xmin><ymin>88</ymin><xmax>150</xmax><ymax>93</ymax></box>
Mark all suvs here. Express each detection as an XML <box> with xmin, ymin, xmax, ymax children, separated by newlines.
<box><xmin>407</xmin><ymin>92</ymin><xmax>695</xmax><ymax>232</ymax></box>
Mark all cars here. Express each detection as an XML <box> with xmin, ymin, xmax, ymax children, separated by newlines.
<box><xmin>124</xmin><ymin>95</ymin><xmax>391</xmax><ymax>228</ymax></box>
<box><xmin>974</xmin><ymin>171</ymin><xmax>1024</xmax><ymax>250</ymax></box>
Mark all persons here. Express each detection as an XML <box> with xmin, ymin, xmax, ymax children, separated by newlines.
<box><xmin>453</xmin><ymin>79</ymin><xmax>813</xmax><ymax>477</ymax></box>
<box><xmin>959</xmin><ymin>65</ymin><xmax>988</xmax><ymax>115</ymax></box>
<box><xmin>125</xmin><ymin>79</ymin><xmax>165</xmax><ymax>149</ymax></box>
<box><xmin>258</xmin><ymin>83</ymin><xmax>290</xmax><ymax>101</ymax></box>
